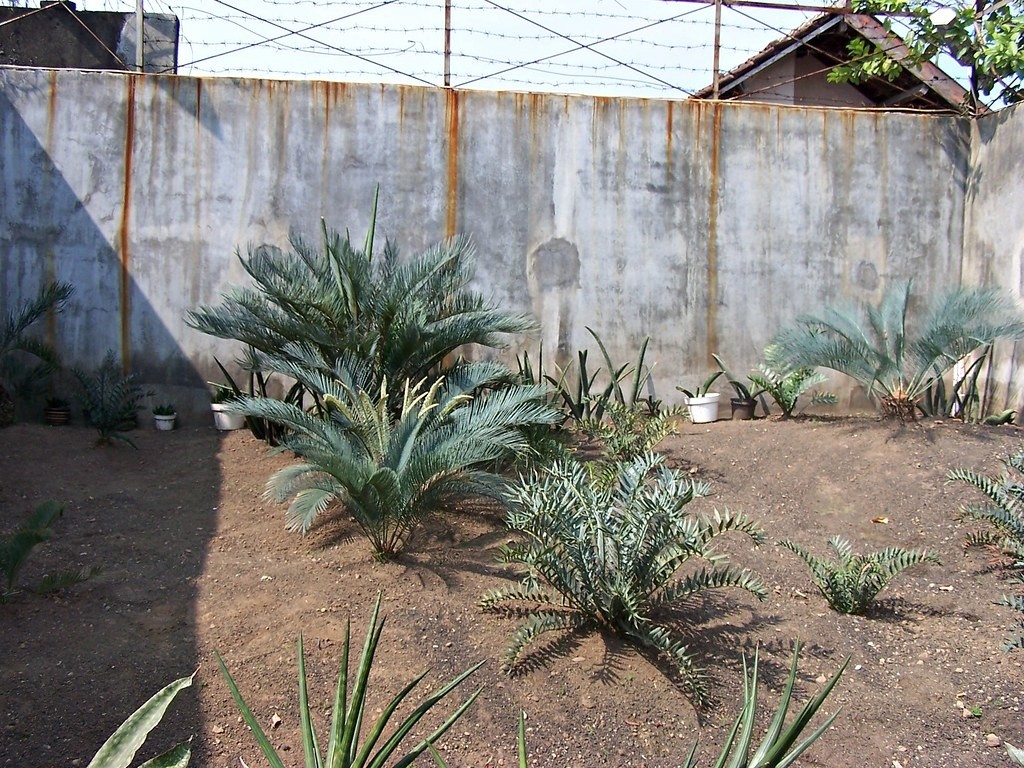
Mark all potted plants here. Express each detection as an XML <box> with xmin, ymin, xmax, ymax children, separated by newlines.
<box><xmin>152</xmin><ymin>403</ymin><xmax>177</xmax><ymax>430</ymax></box>
<box><xmin>711</xmin><ymin>352</ymin><xmax>773</xmax><ymax>419</ymax></box>
<box><xmin>208</xmin><ymin>383</ymin><xmax>244</xmax><ymax>430</ymax></box>
<box><xmin>675</xmin><ymin>370</ymin><xmax>726</xmax><ymax>423</ymax></box>
<box><xmin>42</xmin><ymin>393</ymin><xmax>72</xmax><ymax>426</ymax></box>
